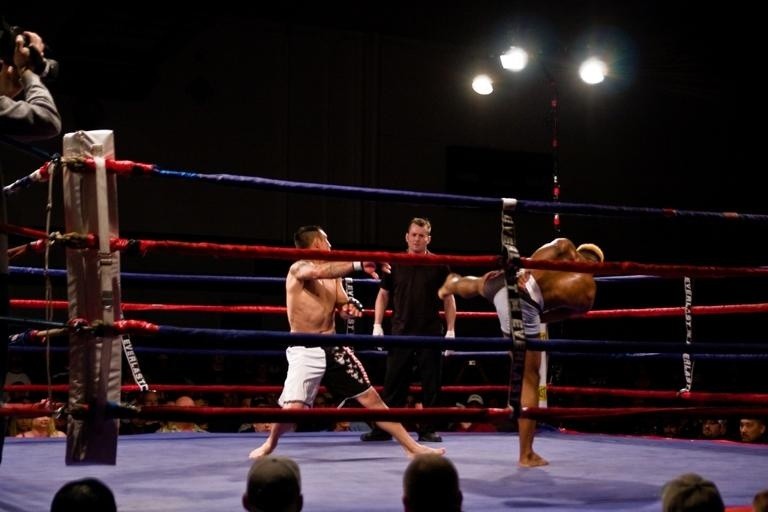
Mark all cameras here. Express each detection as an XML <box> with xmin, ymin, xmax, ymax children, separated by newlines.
<box><xmin>0</xmin><ymin>25</ymin><xmax>60</xmax><ymax>82</ymax></box>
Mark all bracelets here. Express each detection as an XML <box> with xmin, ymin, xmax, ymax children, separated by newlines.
<box><xmin>352</xmin><ymin>260</ymin><xmax>362</xmax><ymax>271</ymax></box>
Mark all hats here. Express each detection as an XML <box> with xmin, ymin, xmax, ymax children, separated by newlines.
<box><xmin>467</xmin><ymin>393</ymin><xmax>485</xmax><ymax>406</ymax></box>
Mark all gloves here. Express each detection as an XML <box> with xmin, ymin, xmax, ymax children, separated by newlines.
<box><xmin>443</xmin><ymin>331</ymin><xmax>459</xmax><ymax>355</ymax></box>
<box><xmin>371</xmin><ymin>323</ymin><xmax>388</xmax><ymax>342</ymax></box>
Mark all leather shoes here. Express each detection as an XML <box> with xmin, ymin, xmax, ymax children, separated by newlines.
<box><xmin>417</xmin><ymin>432</ymin><xmax>443</xmax><ymax>443</ymax></box>
<box><xmin>360</xmin><ymin>430</ymin><xmax>394</xmax><ymax>443</ymax></box>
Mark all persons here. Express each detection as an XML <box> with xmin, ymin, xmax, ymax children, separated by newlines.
<box><xmin>241</xmin><ymin>454</ymin><xmax>304</xmax><ymax>511</ymax></box>
<box><xmin>701</xmin><ymin>416</ymin><xmax>727</xmax><ymax>436</ymax></box>
<box><xmin>0</xmin><ymin>22</ymin><xmax>63</xmax><ymax>143</ymax></box>
<box><xmin>438</xmin><ymin>237</ymin><xmax>604</xmax><ymax>465</ymax></box>
<box><xmin>119</xmin><ymin>389</ymin><xmax>161</xmax><ymax>434</ymax></box>
<box><xmin>402</xmin><ymin>395</ymin><xmax>499</xmax><ymax>433</ymax></box>
<box><xmin>7</xmin><ymin>382</ymin><xmax>67</xmax><ymax>439</ymax></box>
<box><xmin>740</xmin><ymin>419</ymin><xmax>766</xmax><ymax>443</ymax></box>
<box><xmin>334</xmin><ymin>422</ymin><xmax>372</xmax><ymax>432</ymax></box>
<box><xmin>246</xmin><ymin>224</ymin><xmax>446</xmax><ymax>459</ymax></box>
<box><xmin>50</xmin><ymin>475</ymin><xmax>119</xmax><ymax>511</ymax></box>
<box><xmin>154</xmin><ymin>397</ymin><xmax>210</xmax><ymax>433</ymax></box>
<box><xmin>222</xmin><ymin>391</ymin><xmax>297</xmax><ymax>433</ymax></box>
<box><xmin>660</xmin><ymin>472</ymin><xmax>724</xmax><ymax>511</ymax></box>
<box><xmin>313</xmin><ymin>393</ymin><xmax>334</xmax><ymax>408</ymax></box>
<box><xmin>358</xmin><ymin>216</ymin><xmax>457</xmax><ymax>441</ymax></box>
<box><xmin>663</xmin><ymin>415</ymin><xmax>688</xmax><ymax>438</ymax></box>
<box><xmin>401</xmin><ymin>451</ymin><xmax>463</xmax><ymax>511</ymax></box>
<box><xmin>193</xmin><ymin>398</ymin><xmax>208</xmax><ymax>429</ymax></box>
